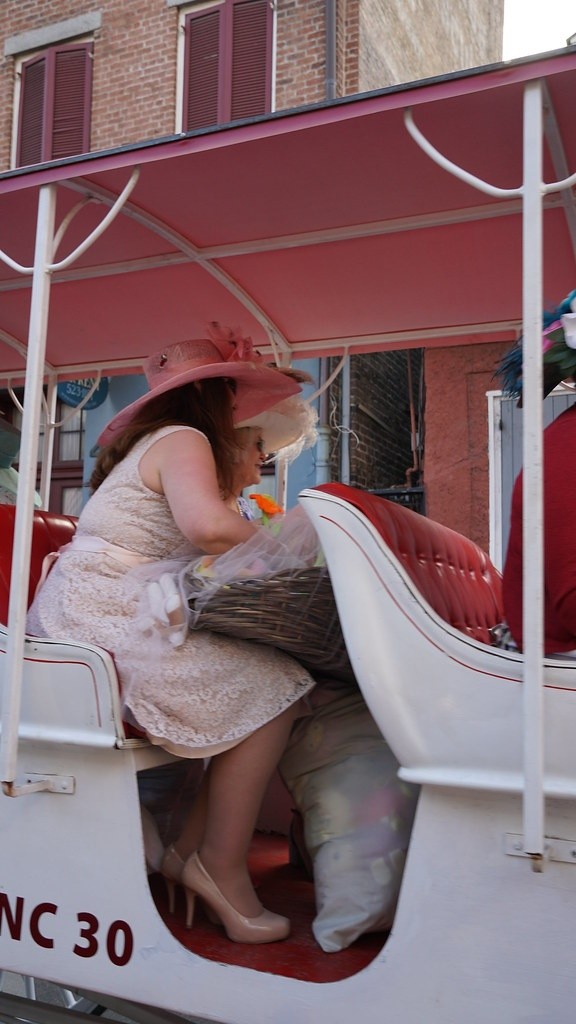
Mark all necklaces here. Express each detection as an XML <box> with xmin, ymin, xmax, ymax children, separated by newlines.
<box><xmin>236</xmin><ymin>501</ymin><xmax>244</xmax><ymax>517</ymax></box>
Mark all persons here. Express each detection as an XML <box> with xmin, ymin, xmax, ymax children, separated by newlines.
<box><xmin>25</xmin><ymin>321</ymin><xmax>316</xmax><ymax>944</ymax></box>
<box><xmin>492</xmin><ymin>290</ymin><xmax>576</xmax><ymax>659</ymax></box>
<box><xmin>222</xmin><ymin>395</ymin><xmax>320</xmax><ymax>521</ymax></box>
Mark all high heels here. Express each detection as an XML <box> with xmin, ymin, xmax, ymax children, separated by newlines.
<box><xmin>287</xmin><ymin>809</ymin><xmax>315</xmax><ymax>879</ymax></box>
<box><xmin>159</xmin><ymin>843</ymin><xmax>224</xmax><ymax>926</ymax></box>
<box><xmin>178</xmin><ymin>849</ymin><xmax>290</xmax><ymax>944</ymax></box>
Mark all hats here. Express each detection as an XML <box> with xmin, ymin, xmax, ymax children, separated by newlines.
<box><xmin>0</xmin><ymin>418</ymin><xmax>42</xmax><ymax>508</ymax></box>
<box><xmin>96</xmin><ymin>318</ymin><xmax>319</xmax><ymax>448</ymax></box>
<box><xmin>233</xmin><ymin>394</ymin><xmax>321</xmax><ymax>465</ymax></box>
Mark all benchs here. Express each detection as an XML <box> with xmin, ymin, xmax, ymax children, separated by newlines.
<box><xmin>0</xmin><ymin>480</ymin><xmax>576</xmax><ymax>797</ymax></box>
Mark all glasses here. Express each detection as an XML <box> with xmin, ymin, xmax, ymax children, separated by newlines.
<box><xmin>243</xmin><ymin>440</ymin><xmax>264</xmax><ymax>452</ymax></box>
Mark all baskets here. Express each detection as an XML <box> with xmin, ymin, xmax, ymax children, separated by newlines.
<box><xmin>189</xmin><ymin>567</ymin><xmax>358</xmax><ymax>686</ymax></box>
